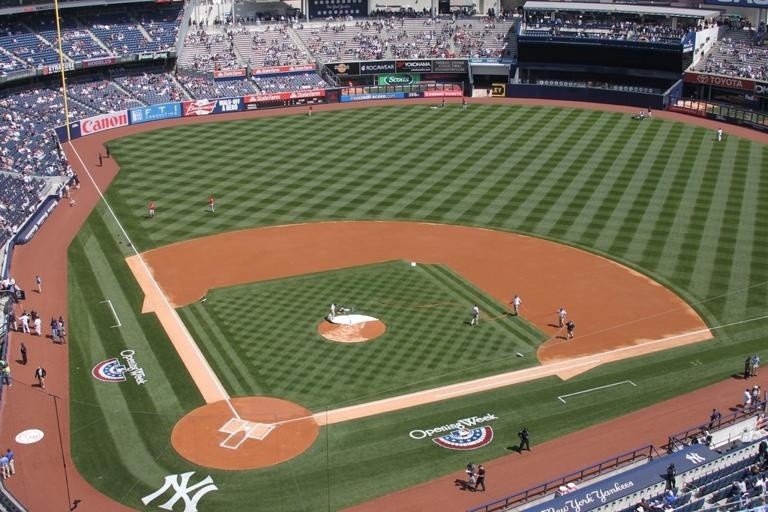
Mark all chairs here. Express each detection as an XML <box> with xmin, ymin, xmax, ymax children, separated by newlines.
<box><xmin>0</xmin><ymin>116</ymin><xmax>75</xmax><ymax>248</ymax></box>
<box><xmin>1</xmin><ymin>21</ymin><xmax>181</xmax><ymax>130</ymax></box>
<box><xmin>618</xmin><ymin>453</ymin><xmax>767</xmax><ymax>511</ymax></box>
